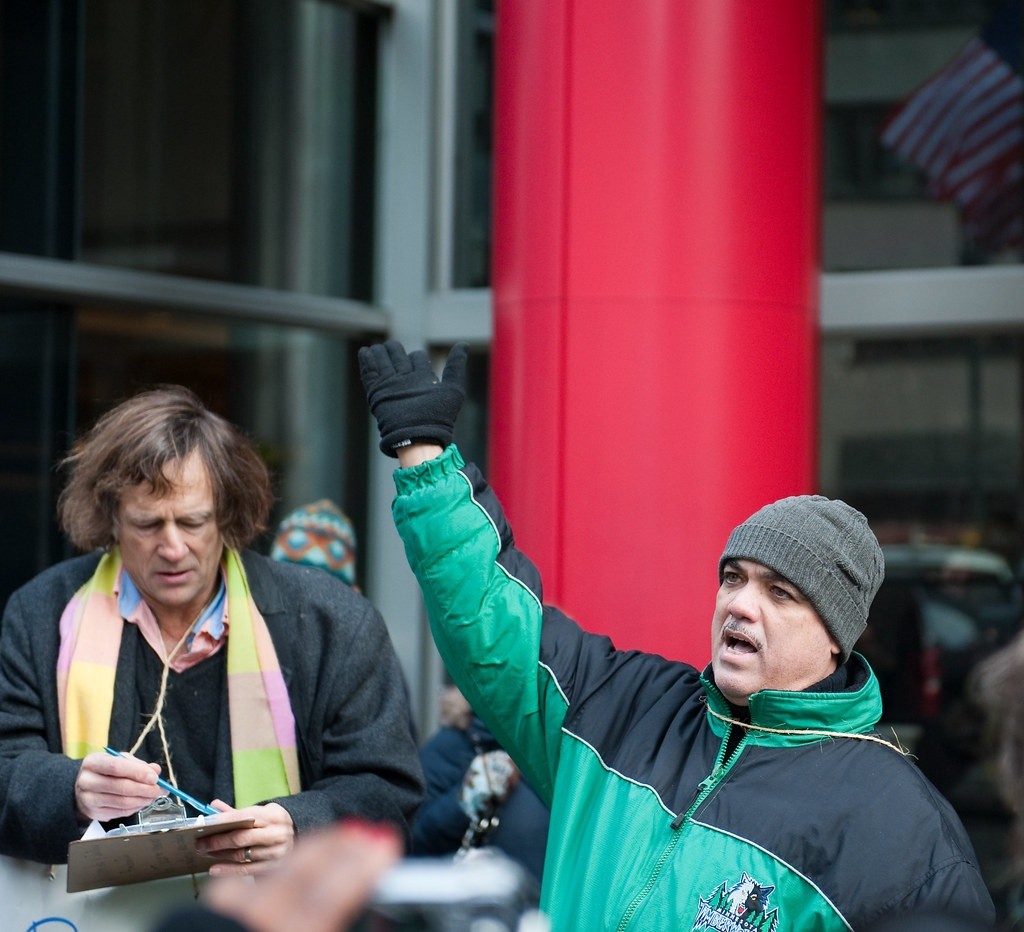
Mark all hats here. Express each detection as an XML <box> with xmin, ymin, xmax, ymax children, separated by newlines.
<box><xmin>718</xmin><ymin>494</ymin><xmax>885</xmax><ymax>665</ymax></box>
<box><xmin>270</xmin><ymin>499</ymin><xmax>357</xmax><ymax>587</ymax></box>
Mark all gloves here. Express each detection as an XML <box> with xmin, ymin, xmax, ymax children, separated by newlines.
<box><xmin>461</xmin><ymin>750</ymin><xmax>520</xmax><ymax>823</ymax></box>
<box><xmin>358</xmin><ymin>340</ymin><xmax>471</xmax><ymax>459</ymax></box>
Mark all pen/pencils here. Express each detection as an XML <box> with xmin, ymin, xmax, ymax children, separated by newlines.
<box><xmin>103</xmin><ymin>745</ymin><xmax>223</xmax><ymax>815</ymax></box>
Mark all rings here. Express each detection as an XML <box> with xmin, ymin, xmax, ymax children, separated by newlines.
<box><xmin>244</xmin><ymin>848</ymin><xmax>252</xmax><ymax>862</ymax></box>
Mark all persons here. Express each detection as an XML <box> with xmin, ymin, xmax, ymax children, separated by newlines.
<box><xmin>356</xmin><ymin>338</ymin><xmax>997</xmax><ymax>932</ymax></box>
<box><xmin>0</xmin><ymin>388</ymin><xmax>550</xmax><ymax>932</ymax></box>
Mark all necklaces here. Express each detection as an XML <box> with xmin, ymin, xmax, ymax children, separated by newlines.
<box><xmin>699</xmin><ymin>695</ymin><xmax>919</xmax><ymax>760</ymax></box>
